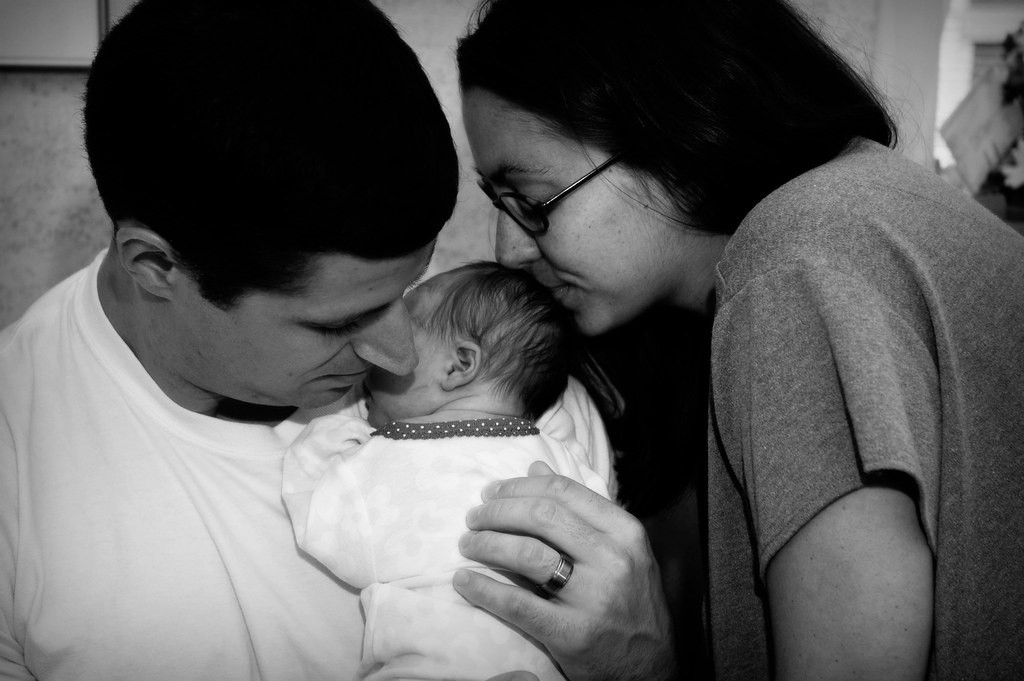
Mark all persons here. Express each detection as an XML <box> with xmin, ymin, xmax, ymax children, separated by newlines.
<box><xmin>0</xmin><ymin>0</ymin><xmax>1024</xmax><ymax>681</ymax></box>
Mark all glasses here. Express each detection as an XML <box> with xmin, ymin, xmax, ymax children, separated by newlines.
<box><xmin>477</xmin><ymin>156</ymin><xmax>618</xmax><ymax>236</ymax></box>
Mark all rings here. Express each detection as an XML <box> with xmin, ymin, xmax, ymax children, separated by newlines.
<box><xmin>536</xmin><ymin>557</ymin><xmax>574</xmax><ymax>600</ymax></box>
